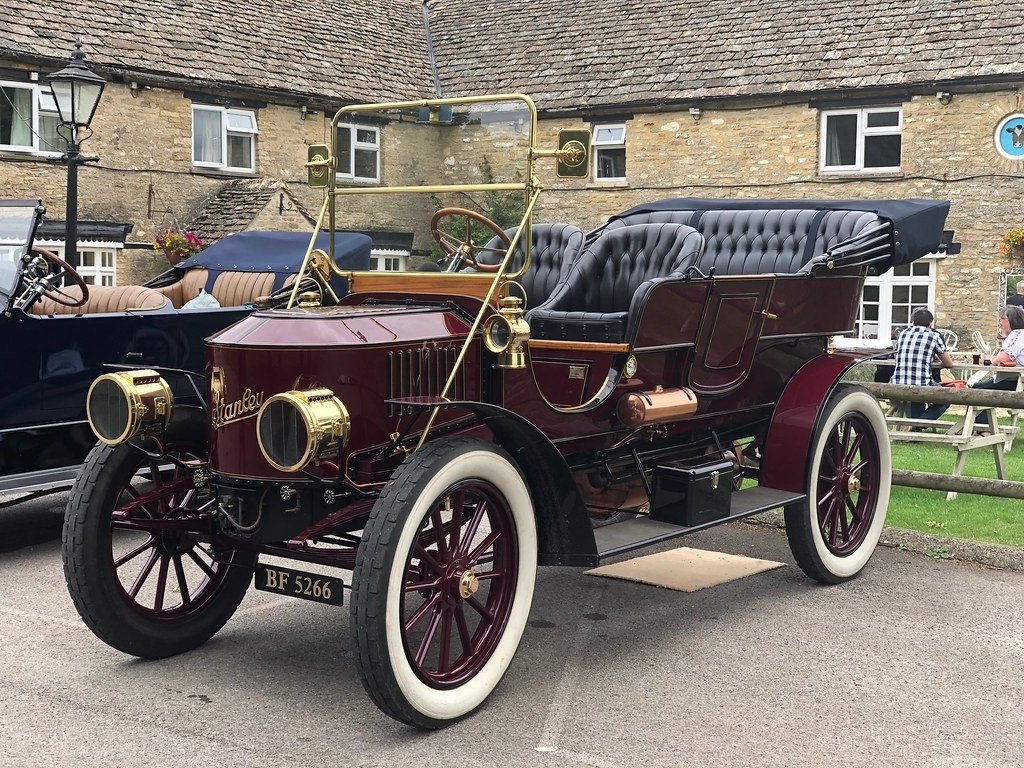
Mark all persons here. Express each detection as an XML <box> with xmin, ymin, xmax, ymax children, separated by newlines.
<box><xmin>886</xmin><ymin>309</ymin><xmax>953</xmax><ymax>443</ymax></box>
<box><xmin>965</xmin><ymin>306</ymin><xmax>1024</xmax><ymax>437</ymax></box>
<box><xmin>1006</xmin><ymin>280</ymin><xmax>1024</xmax><ymax>308</ymax></box>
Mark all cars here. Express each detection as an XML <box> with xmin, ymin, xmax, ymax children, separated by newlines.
<box><xmin>60</xmin><ymin>93</ymin><xmax>962</xmax><ymax>732</ymax></box>
<box><xmin>1</xmin><ymin>194</ymin><xmax>372</xmax><ymax>508</ymax></box>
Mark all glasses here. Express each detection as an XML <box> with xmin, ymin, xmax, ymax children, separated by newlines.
<box><xmin>999</xmin><ymin>317</ymin><xmax>1008</xmax><ymax>321</ymax></box>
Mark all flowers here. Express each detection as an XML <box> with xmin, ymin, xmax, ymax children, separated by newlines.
<box><xmin>152</xmin><ymin>229</ymin><xmax>202</xmax><ymax>259</ymax></box>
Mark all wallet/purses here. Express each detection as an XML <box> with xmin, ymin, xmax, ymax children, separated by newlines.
<box><xmin>1000</xmin><ymin>362</ymin><xmax>1016</xmax><ymax>367</ymax></box>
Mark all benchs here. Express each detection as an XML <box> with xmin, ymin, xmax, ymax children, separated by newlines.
<box><xmin>600</xmin><ymin>194</ymin><xmax>951</xmax><ymax>277</ymax></box>
<box><xmin>153</xmin><ymin>229</ymin><xmax>372</xmax><ymax>308</ymax></box>
<box><xmin>842</xmin><ymin>415</ymin><xmax>1019</xmax><ymax>501</ymax></box>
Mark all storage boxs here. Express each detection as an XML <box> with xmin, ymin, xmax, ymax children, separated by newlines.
<box><xmin>647</xmin><ymin>458</ymin><xmax>734</xmax><ymax>529</ymax></box>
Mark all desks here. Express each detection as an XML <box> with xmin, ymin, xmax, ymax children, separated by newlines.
<box><xmin>855</xmin><ymin>359</ymin><xmax>1024</xmax><ymax>427</ymax></box>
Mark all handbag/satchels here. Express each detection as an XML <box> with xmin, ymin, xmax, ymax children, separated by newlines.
<box><xmin>938</xmin><ymin>380</ymin><xmax>967</xmax><ymax>388</ymax></box>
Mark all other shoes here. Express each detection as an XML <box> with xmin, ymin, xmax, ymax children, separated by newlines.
<box><xmin>977</xmin><ymin>432</ymin><xmax>991</xmax><ymax>437</ymax></box>
<box><xmin>907</xmin><ymin>427</ymin><xmax>923</xmax><ymax>443</ymax></box>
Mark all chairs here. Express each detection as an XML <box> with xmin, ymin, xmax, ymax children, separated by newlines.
<box><xmin>25</xmin><ymin>284</ymin><xmax>175</xmax><ymax>314</ymax></box>
<box><xmin>972</xmin><ymin>330</ymin><xmax>988</xmax><ymax>353</ymax></box>
<box><xmin>459</xmin><ymin>221</ymin><xmax>588</xmax><ymax>324</ymax></box>
<box><xmin>522</xmin><ymin>221</ymin><xmax>706</xmax><ymax>351</ymax></box>
<box><xmin>935</xmin><ymin>329</ymin><xmax>958</xmax><ymax>352</ymax></box>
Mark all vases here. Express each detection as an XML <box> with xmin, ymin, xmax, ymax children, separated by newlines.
<box><xmin>165</xmin><ymin>247</ymin><xmax>183</xmax><ymax>267</ymax></box>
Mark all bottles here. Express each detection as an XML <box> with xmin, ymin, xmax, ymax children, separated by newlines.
<box><xmin>984</xmin><ymin>342</ymin><xmax>991</xmax><ymax>365</ymax></box>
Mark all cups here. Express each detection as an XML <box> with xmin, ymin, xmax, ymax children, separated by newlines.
<box><xmin>972</xmin><ymin>349</ymin><xmax>980</xmax><ymax>365</ymax></box>
